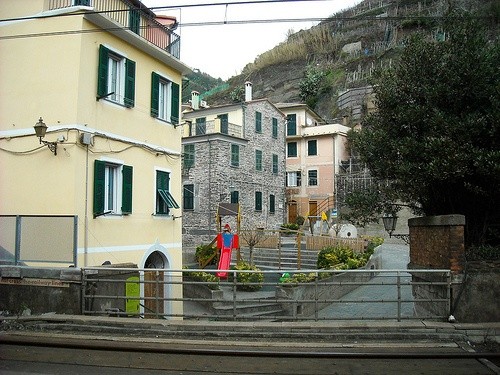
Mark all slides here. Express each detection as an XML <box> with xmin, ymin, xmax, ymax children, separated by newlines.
<box><xmin>216</xmin><ymin>238</ymin><xmax>232</xmax><ymax>278</ymax></box>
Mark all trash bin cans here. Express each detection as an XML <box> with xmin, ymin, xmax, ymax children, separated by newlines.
<box><xmin>85</xmin><ymin>263</ymin><xmax>141</xmax><ymax>319</ymax></box>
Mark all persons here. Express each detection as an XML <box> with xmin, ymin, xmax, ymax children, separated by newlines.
<box><xmin>223</xmin><ymin>224</ymin><xmax>232</xmax><ymax>234</ymax></box>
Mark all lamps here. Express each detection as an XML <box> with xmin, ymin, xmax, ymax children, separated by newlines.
<box><xmin>381</xmin><ymin>206</ymin><xmax>411</xmax><ymax>245</ymax></box>
<box><xmin>33</xmin><ymin>116</ymin><xmax>58</xmax><ymax>157</ymax></box>
